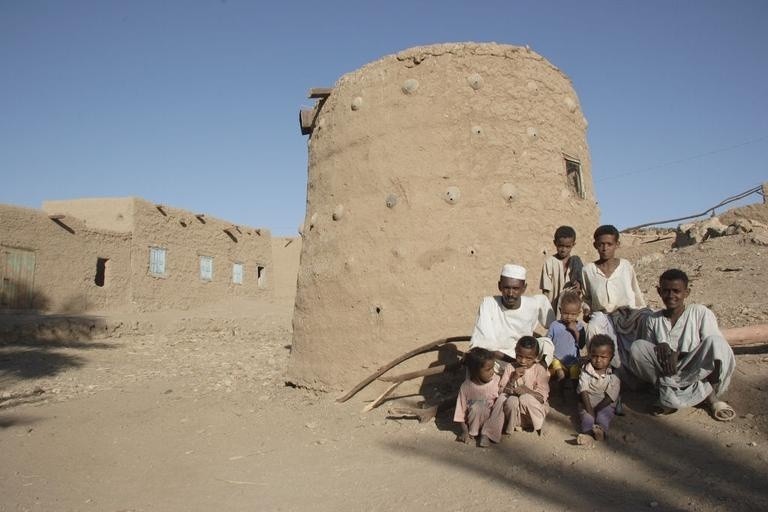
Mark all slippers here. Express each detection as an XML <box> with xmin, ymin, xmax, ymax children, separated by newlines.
<box><xmin>710</xmin><ymin>401</ymin><xmax>736</xmax><ymax>420</ymax></box>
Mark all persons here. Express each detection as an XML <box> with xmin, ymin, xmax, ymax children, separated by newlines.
<box><xmin>539</xmin><ymin>224</ymin><xmax>585</xmax><ymax>302</ymax></box>
<box><xmin>497</xmin><ymin>333</ymin><xmax>552</xmax><ymax>435</ymax></box>
<box><xmin>574</xmin><ymin>330</ymin><xmax>623</xmax><ymax>447</ymax></box>
<box><xmin>544</xmin><ymin>292</ymin><xmax>584</xmax><ymax>404</ymax></box>
<box><xmin>472</xmin><ymin>263</ymin><xmax>555</xmax><ymax>372</ymax></box>
<box><xmin>580</xmin><ymin>223</ymin><xmax>648</xmax><ymax>310</ymax></box>
<box><xmin>451</xmin><ymin>348</ymin><xmax>501</xmax><ymax>447</ymax></box>
<box><xmin>629</xmin><ymin>268</ymin><xmax>739</xmax><ymax>421</ymax></box>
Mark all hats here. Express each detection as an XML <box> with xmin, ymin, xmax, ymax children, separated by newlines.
<box><xmin>502</xmin><ymin>265</ymin><xmax>526</xmax><ymax>280</ymax></box>
